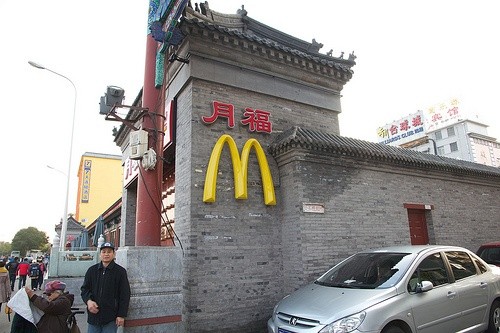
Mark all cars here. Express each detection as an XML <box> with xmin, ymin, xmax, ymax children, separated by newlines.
<box><xmin>266</xmin><ymin>245</ymin><xmax>500</xmax><ymax>333</ymax></box>
<box><xmin>475</xmin><ymin>242</ymin><xmax>500</xmax><ymax>266</ymax></box>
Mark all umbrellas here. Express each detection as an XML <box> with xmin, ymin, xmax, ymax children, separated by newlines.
<box><xmin>71</xmin><ymin>229</ymin><xmax>88</xmax><ymax>251</ymax></box>
<box><xmin>92</xmin><ymin>215</ymin><xmax>106</xmax><ymax>246</ymax></box>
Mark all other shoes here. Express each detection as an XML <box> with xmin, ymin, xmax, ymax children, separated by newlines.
<box><xmin>38</xmin><ymin>287</ymin><xmax>41</xmax><ymax>290</ymax></box>
<box><xmin>34</xmin><ymin>288</ymin><xmax>36</xmax><ymax>291</ymax></box>
<box><xmin>11</xmin><ymin>289</ymin><xmax>14</xmax><ymax>291</ymax></box>
<box><xmin>32</xmin><ymin>289</ymin><xmax>34</xmax><ymax>291</ymax></box>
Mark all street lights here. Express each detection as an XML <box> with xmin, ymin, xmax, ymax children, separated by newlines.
<box><xmin>46</xmin><ymin>164</ymin><xmax>76</xmax><ymax>185</ymax></box>
<box><xmin>28</xmin><ymin>61</ymin><xmax>77</xmax><ymax>252</ymax></box>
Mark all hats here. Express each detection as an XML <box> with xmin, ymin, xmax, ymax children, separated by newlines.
<box><xmin>100</xmin><ymin>242</ymin><xmax>115</xmax><ymax>249</ymax></box>
<box><xmin>42</xmin><ymin>280</ymin><xmax>67</xmax><ymax>294</ymax></box>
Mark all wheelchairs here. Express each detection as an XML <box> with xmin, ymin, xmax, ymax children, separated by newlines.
<box><xmin>66</xmin><ymin>308</ymin><xmax>85</xmax><ymax>333</ymax></box>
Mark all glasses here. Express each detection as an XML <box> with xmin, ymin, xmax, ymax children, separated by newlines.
<box><xmin>46</xmin><ymin>293</ymin><xmax>52</xmax><ymax>296</ymax></box>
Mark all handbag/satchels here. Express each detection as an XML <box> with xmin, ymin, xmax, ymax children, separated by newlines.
<box><xmin>5</xmin><ymin>305</ymin><xmax>12</xmax><ymax>314</ymax></box>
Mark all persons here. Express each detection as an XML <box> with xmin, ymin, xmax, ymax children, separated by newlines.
<box><xmin>11</xmin><ymin>280</ymin><xmax>74</xmax><ymax>333</ymax></box>
<box><xmin>80</xmin><ymin>243</ymin><xmax>130</xmax><ymax>333</ymax></box>
<box><xmin>5</xmin><ymin>257</ymin><xmax>47</xmax><ymax>291</ymax></box>
<box><xmin>0</xmin><ymin>262</ymin><xmax>11</xmax><ymax>312</ymax></box>
